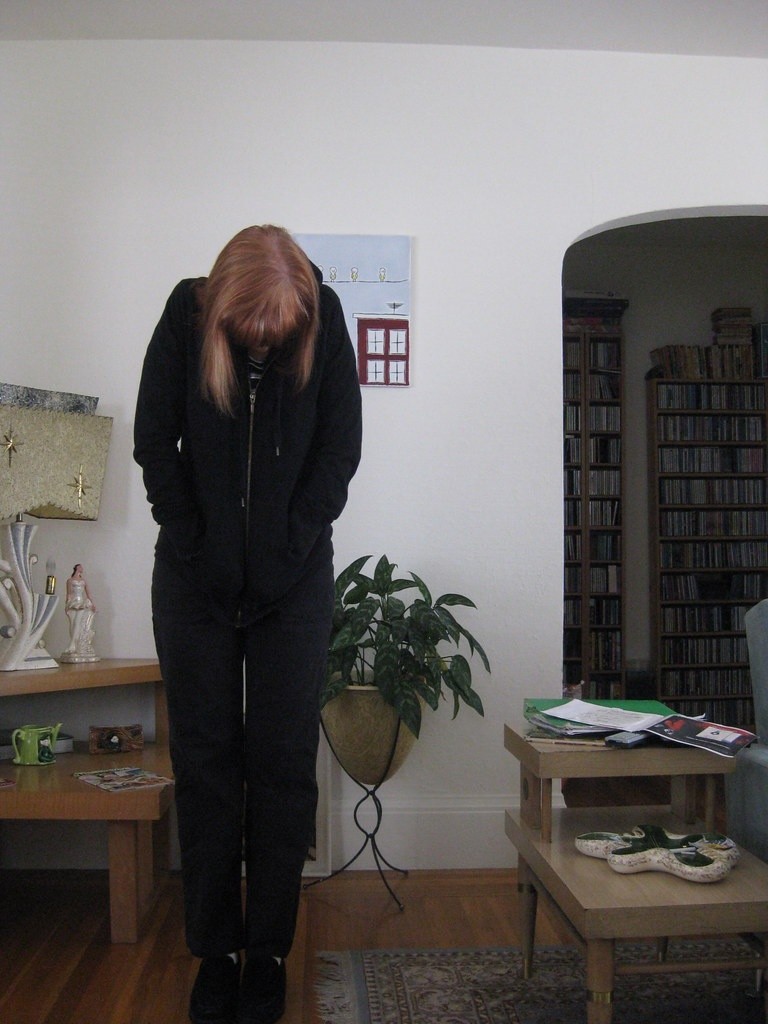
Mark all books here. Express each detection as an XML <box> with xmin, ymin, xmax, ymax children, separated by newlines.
<box><xmin>524</xmin><ymin>728</ymin><xmax>606</xmax><ymax>746</ymax></box>
<box><xmin>563</xmin><ymin>299</ymin><xmax>768</xmax><ymax>725</ymax></box>
<box><xmin>0</xmin><ymin>728</ymin><xmax>74</xmax><ymax>759</ymax></box>
<box><xmin>71</xmin><ymin>766</ymin><xmax>175</xmax><ymax>791</ymax></box>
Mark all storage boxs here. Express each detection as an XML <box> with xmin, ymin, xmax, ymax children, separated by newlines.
<box><xmin>562</xmin><ymin>288</ymin><xmax>629</xmax><ymax>333</ymax></box>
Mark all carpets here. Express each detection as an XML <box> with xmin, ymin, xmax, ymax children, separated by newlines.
<box><xmin>311</xmin><ymin>936</ymin><xmax>768</xmax><ymax>1024</ymax></box>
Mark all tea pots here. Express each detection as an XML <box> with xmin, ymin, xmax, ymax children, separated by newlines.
<box><xmin>11</xmin><ymin>722</ymin><xmax>63</xmax><ymax>765</ymax></box>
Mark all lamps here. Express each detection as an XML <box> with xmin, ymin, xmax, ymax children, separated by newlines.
<box><xmin>0</xmin><ymin>382</ymin><xmax>112</xmax><ymax>673</ymax></box>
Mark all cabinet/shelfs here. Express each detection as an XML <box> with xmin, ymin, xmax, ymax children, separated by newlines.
<box><xmin>649</xmin><ymin>378</ymin><xmax>766</xmax><ymax>750</ymax></box>
<box><xmin>502</xmin><ymin>722</ymin><xmax>768</xmax><ymax>1024</ymax></box>
<box><xmin>563</xmin><ymin>330</ymin><xmax>626</xmax><ymax>702</ymax></box>
<box><xmin>0</xmin><ymin>658</ymin><xmax>175</xmax><ymax>942</ymax></box>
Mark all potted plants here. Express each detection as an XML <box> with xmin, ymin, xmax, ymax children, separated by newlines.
<box><xmin>321</xmin><ymin>555</ymin><xmax>491</xmax><ymax>786</ymax></box>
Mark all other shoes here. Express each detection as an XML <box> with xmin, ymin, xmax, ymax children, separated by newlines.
<box><xmin>235</xmin><ymin>956</ymin><xmax>286</xmax><ymax>1024</ymax></box>
<box><xmin>189</xmin><ymin>951</ymin><xmax>242</xmax><ymax>1024</ymax></box>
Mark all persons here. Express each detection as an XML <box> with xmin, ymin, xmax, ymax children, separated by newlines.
<box><xmin>66</xmin><ymin>564</ymin><xmax>96</xmax><ymax>653</ymax></box>
<box><xmin>134</xmin><ymin>225</ymin><xmax>362</xmax><ymax>1024</ymax></box>
